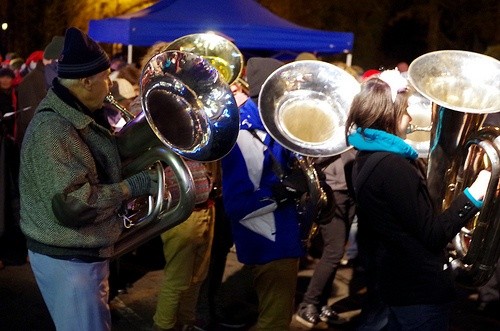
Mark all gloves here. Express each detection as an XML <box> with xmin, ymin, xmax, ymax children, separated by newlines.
<box><xmin>273</xmin><ymin>167</ymin><xmax>307</xmax><ymax>202</ymax></box>
<box><xmin>124</xmin><ymin>172</ymin><xmax>158</xmax><ymax>200</ymax></box>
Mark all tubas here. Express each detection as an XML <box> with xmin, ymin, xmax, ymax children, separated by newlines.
<box><xmin>165</xmin><ymin>32</ymin><xmax>243</xmax><ymax>87</ymax></box>
<box><xmin>102</xmin><ymin>50</ymin><xmax>239</xmax><ymax>257</ymax></box>
<box><xmin>408</xmin><ymin>49</ymin><xmax>500</xmax><ymax>286</ymax></box>
<box><xmin>259</xmin><ymin>59</ymin><xmax>364</xmax><ymax>243</ymax></box>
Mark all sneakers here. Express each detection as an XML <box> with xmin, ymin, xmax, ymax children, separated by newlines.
<box><xmin>295</xmin><ymin>302</ymin><xmax>343</xmax><ymax>328</ymax></box>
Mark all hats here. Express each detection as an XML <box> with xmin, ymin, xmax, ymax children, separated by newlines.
<box><xmin>57</xmin><ymin>26</ymin><xmax>112</xmax><ymax>80</ymax></box>
<box><xmin>0</xmin><ymin>68</ymin><xmax>15</xmax><ymax>78</ymax></box>
<box><xmin>246</xmin><ymin>57</ymin><xmax>285</xmax><ymax>99</ymax></box>
<box><xmin>44</xmin><ymin>35</ymin><xmax>64</xmax><ymax>59</ymax></box>
<box><xmin>26</xmin><ymin>51</ymin><xmax>44</xmax><ymax>64</ymax></box>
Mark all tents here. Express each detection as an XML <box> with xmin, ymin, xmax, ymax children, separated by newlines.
<box><xmin>90</xmin><ymin>0</ymin><xmax>354</xmax><ymax>52</ymax></box>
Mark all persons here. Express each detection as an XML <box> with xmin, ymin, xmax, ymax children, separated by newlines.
<box><xmin>343</xmin><ymin>77</ymin><xmax>500</xmax><ymax>331</ymax></box>
<box><xmin>20</xmin><ymin>26</ymin><xmax>163</xmax><ymax>331</ymax></box>
<box><xmin>0</xmin><ymin>53</ymin><xmax>499</xmax><ymax>331</ymax></box>
